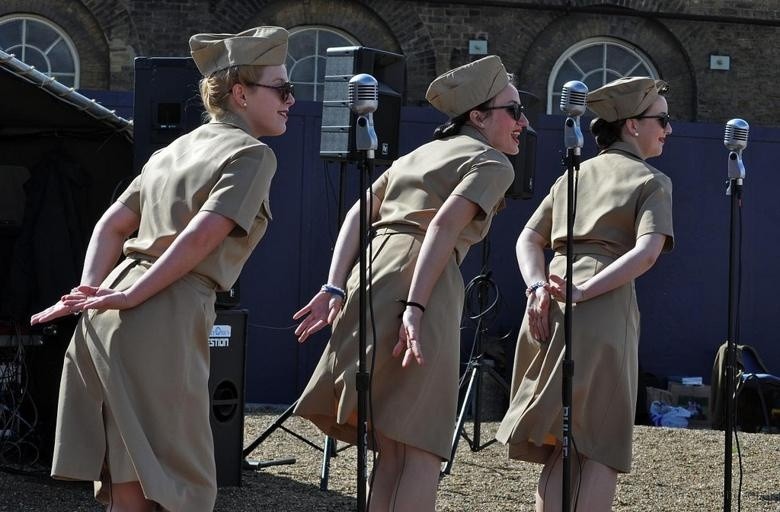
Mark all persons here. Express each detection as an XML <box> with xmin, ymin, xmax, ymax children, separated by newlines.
<box><xmin>291</xmin><ymin>55</ymin><xmax>530</xmax><ymax>511</ymax></box>
<box><xmin>494</xmin><ymin>75</ymin><xmax>676</xmax><ymax>511</ymax></box>
<box><xmin>30</xmin><ymin>26</ymin><xmax>297</xmax><ymax>512</ymax></box>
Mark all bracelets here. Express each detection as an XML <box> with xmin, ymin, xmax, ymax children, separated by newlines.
<box><xmin>393</xmin><ymin>299</ymin><xmax>426</xmax><ymax>312</ymax></box>
<box><xmin>319</xmin><ymin>285</ymin><xmax>348</xmax><ymax>303</ymax></box>
<box><xmin>525</xmin><ymin>281</ymin><xmax>546</xmax><ymax>295</ymax></box>
<box><xmin>68</xmin><ymin>286</ymin><xmax>87</xmax><ymax>316</ymax></box>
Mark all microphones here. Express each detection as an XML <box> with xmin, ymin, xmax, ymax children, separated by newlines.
<box><xmin>347</xmin><ymin>74</ymin><xmax>378</xmax><ymax>127</ymax></box>
<box><xmin>722</xmin><ymin>118</ymin><xmax>750</xmax><ymax>160</ymax></box>
<box><xmin>560</xmin><ymin>80</ymin><xmax>587</xmax><ymax>126</ymax></box>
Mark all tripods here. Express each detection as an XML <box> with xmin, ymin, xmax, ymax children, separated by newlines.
<box><xmin>443</xmin><ymin>236</ymin><xmax>510</xmax><ymax>474</ymax></box>
<box><xmin>242</xmin><ymin>164</ymin><xmax>337</xmax><ymax>491</ymax></box>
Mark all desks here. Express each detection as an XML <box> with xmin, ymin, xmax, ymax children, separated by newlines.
<box><xmin>670</xmin><ymin>383</ymin><xmax>711</xmax><ymax>423</ymax></box>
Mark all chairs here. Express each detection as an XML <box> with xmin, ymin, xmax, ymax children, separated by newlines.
<box><xmin>646</xmin><ymin>386</ymin><xmax>672</xmax><ymax>412</ymax></box>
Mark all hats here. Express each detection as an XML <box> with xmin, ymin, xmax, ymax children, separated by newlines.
<box><xmin>587</xmin><ymin>76</ymin><xmax>658</xmax><ymax>122</ymax></box>
<box><xmin>189</xmin><ymin>26</ymin><xmax>289</xmax><ymax>77</ymax></box>
<box><xmin>425</xmin><ymin>55</ymin><xmax>509</xmax><ymax>119</ymax></box>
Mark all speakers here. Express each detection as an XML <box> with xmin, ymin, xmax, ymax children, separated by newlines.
<box><xmin>132</xmin><ymin>57</ymin><xmax>209</xmax><ymax>179</ymax></box>
<box><xmin>209</xmin><ymin>309</ymin><xmax>247</xmax><ymax>488</ymax></box>
<box><xmin>505</xmin><ymin>90</ymin><xmax>539</xmax><ymax>200</ymax></box>
<box><xmin>319</xmin><ymin>45</ymin><xmax>406</xmax><ymax>162</ymax></box>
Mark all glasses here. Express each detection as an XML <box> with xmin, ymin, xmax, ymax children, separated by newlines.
<box><xmin>621</xmin><ymin>114</ymin><xmax>671</xmax><ymax>128</ymax></box>
<box><xmin>488</xmin><ymin>104</ymin><xmax>524</xmax><ymax>120</ymax></box>
<box><xmin>229</xmin><ymin>82</ymin><xmax>295</xmax><ymax>100</ymax></box>
